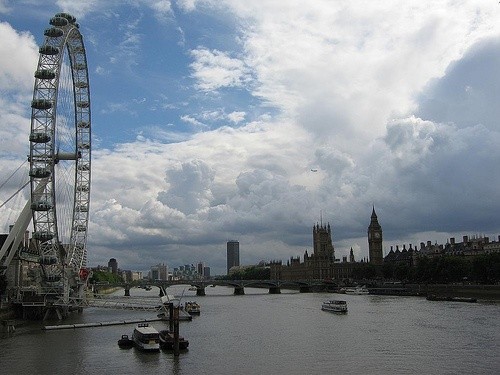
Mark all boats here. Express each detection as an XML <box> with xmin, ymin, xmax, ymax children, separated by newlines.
<box><xmin>185</xmin><ymin>299</ymin><xmax>200</xmax><ymax>313</ymax></box>
<box><xmin>322</xmin><ymin>299</ymin><xmax>347</xmax><ymax>312</ymax></box>
<box><xmin>428</xmin><ymin>293</ymin><xmax>475</xmax><ymax>301</ymax></box>
<box><xmin>160</xmin><ymin>302</ymin><xmax>190</xmax><ymax>354</ymax></box>
<box><xmin>118</xmin><ymin>335</ymin><xmax>133</xmax><ymax>346</ymax></box>
<box><xmin>346</xmin><ymin>285</ymin><xmax>368</xmax><ymax>295</ymax></box>
<box><xmin>133</xmin><ymin>323</ymin><xmax>161</xmax><ymax>351</ymax></box>
